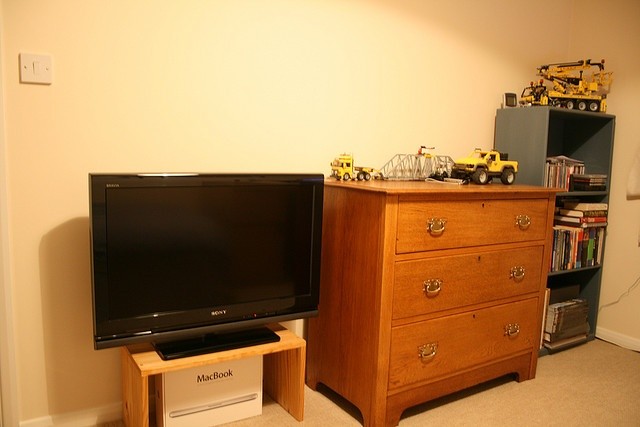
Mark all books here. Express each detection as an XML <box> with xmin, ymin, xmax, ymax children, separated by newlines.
<box><xmin>544</xmin><ymin>155</ymin><xmax>585</xmax><ymax>192</ymax></box>
<box><xmin>543</xmin><ymin>283</ymin><xmax>591</xmax><ymax>351</ymax></box>
<box><xmin>548</xmin><ymin>225</ymin><xmax>605</xmax><ymax>272</ymax></box>
<box><xmin>569</xmin><ymin>173</ymin><xmax>607</xmax><ymax>190</ymax></box>
<box><xmin>553</xmin><ymin>201</ymin><xmax>608</xmax><ymax>228</ymax></box>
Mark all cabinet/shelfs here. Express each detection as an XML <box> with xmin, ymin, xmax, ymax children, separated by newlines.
<box><xmin>303</xmin><ymin>185</ymin><xmax>567</xmax><ymax>427</ymax></box>
<box><xmin>493</xmin><ymin>109</ymin><xmax>615</xmax><ymax>357</ymax></box>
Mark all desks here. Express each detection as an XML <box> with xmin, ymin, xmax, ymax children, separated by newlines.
<box><xmin>121</xmin><ymin>322</ymin><xmax>306</xmax><ymax>427</ymax></box>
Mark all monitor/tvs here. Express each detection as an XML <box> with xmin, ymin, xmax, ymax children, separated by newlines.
<box><xmin>88</xmin><ymin>170</ymin><xmax>325</xmax><ymax>362</ymax></box>
